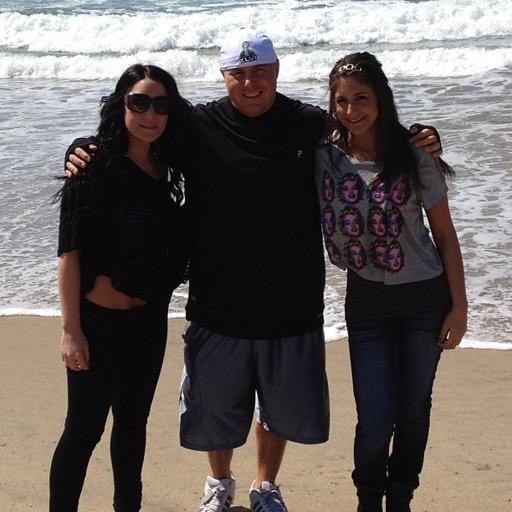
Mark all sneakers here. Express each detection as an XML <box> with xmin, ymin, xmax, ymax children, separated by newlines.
<box><xmin>249</xmin><ymin>479</ymin><xmax>286</xmax><ymax>512</ymax></box>
<box><xmin>198</xmin><ymin>471</ymin><xmax>236</xmax><ymax>512</ymax></box>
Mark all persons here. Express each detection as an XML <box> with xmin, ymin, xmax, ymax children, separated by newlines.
<box><xmin>311</xmin><ymin>52</ymin><xmax>466</xmax><ymax>512</ymax></box>
<box><xmin>65</xmin><ymin>31</ymin><xmax>444</xmax><ymax>511</ymax></box>
<box><xmin>49</xmin><ymin>32</ymin><xmax>467</xmax><ymax>512</ymax></box>
<box><xmin>49</xmin><ymin>62</ymin><xmax>194</xmax><ymax>511</ymax></box>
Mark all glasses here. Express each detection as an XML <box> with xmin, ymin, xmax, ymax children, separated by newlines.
<box><xmin>118</xmin><ymin>94</ymin><xmax>173</xmax><ymax>116</ymax></box>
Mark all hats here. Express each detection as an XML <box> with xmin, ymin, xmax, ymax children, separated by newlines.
<box><xmin>219</xmin><ymin>28</ymin><xmax>277</xmax><ymax>69</ymax></box>
